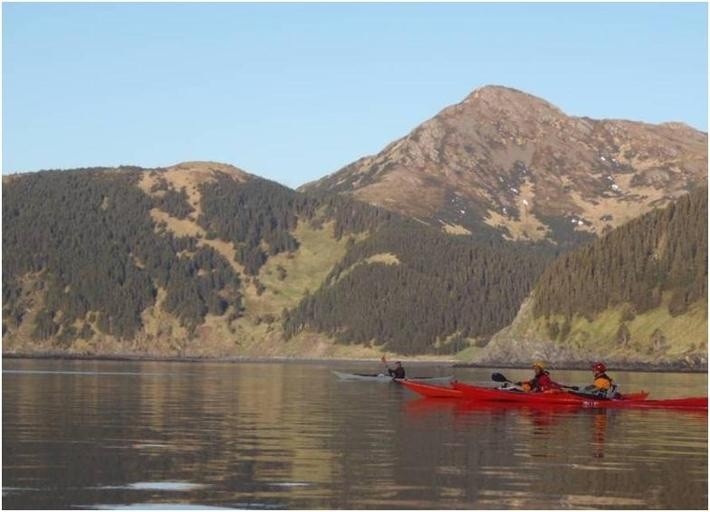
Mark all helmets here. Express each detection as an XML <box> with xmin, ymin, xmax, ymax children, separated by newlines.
<box><xmin>592</xmin><ymin>362</ymin><xmax>606</xmax><ymax>377</ymax></box>
<box><xmin>532</xmin><ymin>361</ymin><xmax>545</xmax><ymax>370</ymax></box>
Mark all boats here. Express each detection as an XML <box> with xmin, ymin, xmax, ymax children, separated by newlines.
<box><xmin>447</xmin><ymin>380</ymin><xmax>708</xmax><ymax>412</ymax></box>
<box><xmin>331</xmin><ymin>367</ymin><xmax>435</xmax><ymax>382</ymax></box>
<box><xmin>394</xmin><ymin>377</ymin><xmax>648</xmax><ymax>411</ymax></box>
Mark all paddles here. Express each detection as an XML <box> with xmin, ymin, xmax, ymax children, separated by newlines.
<box><xmin>492</xmin><ymin>373</ymin><xmax>522</xmax><ymax>385</ymax></box>
<box><xmin>554</xmin><ymin>382</ymin><xmax>579</xmax><ymax>391</ymax></box>
<box><xmin>381</xmin><ymin>355</ymin><xmax>393</xmax><ymax>377</ymax></box>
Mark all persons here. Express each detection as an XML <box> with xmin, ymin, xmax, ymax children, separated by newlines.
<box><xmin>519</xmin><ymin>361</ymin><xmax>552</xmax><ymax>392</ymax></box>
<box><xmin>386</xmin><ymin>361</ymin><xmax>404</xmax><ymax>378</ymax></box>
<box><xmin>589</xmin><ymin>362</ymin><xmax>614</xmax><ymax>397</ymax></box>
